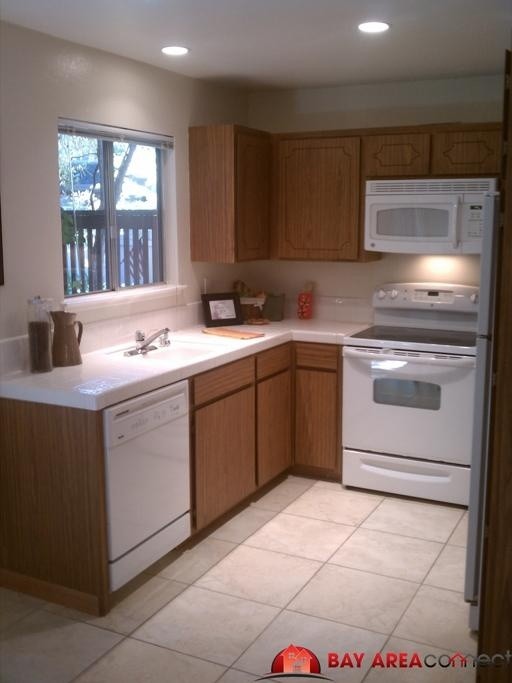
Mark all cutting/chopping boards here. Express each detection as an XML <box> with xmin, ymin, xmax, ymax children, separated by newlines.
<box><xmin>200</xmin><ymin>326</ymin><xmax>266</xmax><ymax>340</ymax></box>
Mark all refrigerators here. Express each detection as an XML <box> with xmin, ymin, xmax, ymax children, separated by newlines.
<box><xmin>460</xmin><ymin>191</ymin><xmax>503</xmax><ymax>634</ymax></box>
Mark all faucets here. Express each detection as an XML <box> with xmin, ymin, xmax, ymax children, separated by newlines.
<box><xmin>136</xmin><ymin>328</ymin><xmax>172</xmax><ymax>352</ymax></box>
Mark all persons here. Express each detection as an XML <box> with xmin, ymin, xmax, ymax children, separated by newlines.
<box><xmin>211</xmin><ymin>301</ymin><xmax>226</xmax><ymax>320</ymax></box>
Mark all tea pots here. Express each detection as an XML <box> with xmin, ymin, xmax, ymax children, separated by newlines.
<box><xmin>48</xmin><ymin>309</ymin><xmax>84</xmax><ymax>367</ymax></box>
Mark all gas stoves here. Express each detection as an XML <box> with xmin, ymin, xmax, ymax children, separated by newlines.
<box><xmin>339</xmin><ymin>325</ymin><xmax>478</xmax><ymax>357</ymax></box>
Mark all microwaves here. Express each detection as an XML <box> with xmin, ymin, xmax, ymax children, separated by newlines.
<box><xmin>362</xmin><ymin>174</ymin><xmax>497</xmax><ymax>254</ymax></box>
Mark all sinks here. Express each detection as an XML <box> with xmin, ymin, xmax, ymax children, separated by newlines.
<box><xmin>142</xmin><ymin>346</ymin><xmax>212</xmax><ymax>365</ymax></box>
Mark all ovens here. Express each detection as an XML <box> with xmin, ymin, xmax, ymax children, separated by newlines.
<box><xmin>339</xmin><ymin>347</ymin><xmax>478</xmax><ymax>510</ymax></box>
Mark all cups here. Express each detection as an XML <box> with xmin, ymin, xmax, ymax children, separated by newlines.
<box><xmin>261</xmin><ymin>294</ymin><xmax>286</xmax><ymax>322</ymax></box>
<box><xmin>298</xmin><ymin>291</ymin><xmax>314</xmax><ymax>320</ymax></box>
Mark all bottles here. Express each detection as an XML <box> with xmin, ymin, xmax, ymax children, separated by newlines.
<box><xmin>26</xmin><ymin>294</ymin><xmax>53</xmax><ymax>372</ymax></box>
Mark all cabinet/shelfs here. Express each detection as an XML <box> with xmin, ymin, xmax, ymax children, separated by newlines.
<box><xmin>192</xmin><ymin>339</ymin><xmax>293</xmax><ymax>537</ymax></box>
<box><xmin>360</xmin><ymin>120</ymin><xmax>504</xmax><ymax>177</ymax></box>
<box><xmin>272</xmin><ymin>126</ymin><xmax>384</xmax><ymax>262</ymax></box>
<box><xmin>187</xmin><ymin>123</ymin><xmax>271</xmax><ymax>264</ymax></box>
<box><xmin>293</xmin><ymin>340</ymin><xmax>343</xmax><ymax>486</ymax></box>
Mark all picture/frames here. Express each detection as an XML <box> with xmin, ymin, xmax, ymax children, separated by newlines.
<box><xmin>201</xmin><ymin>291</ymin><xmax>245</xmax><ymax>327</ymax></box>
